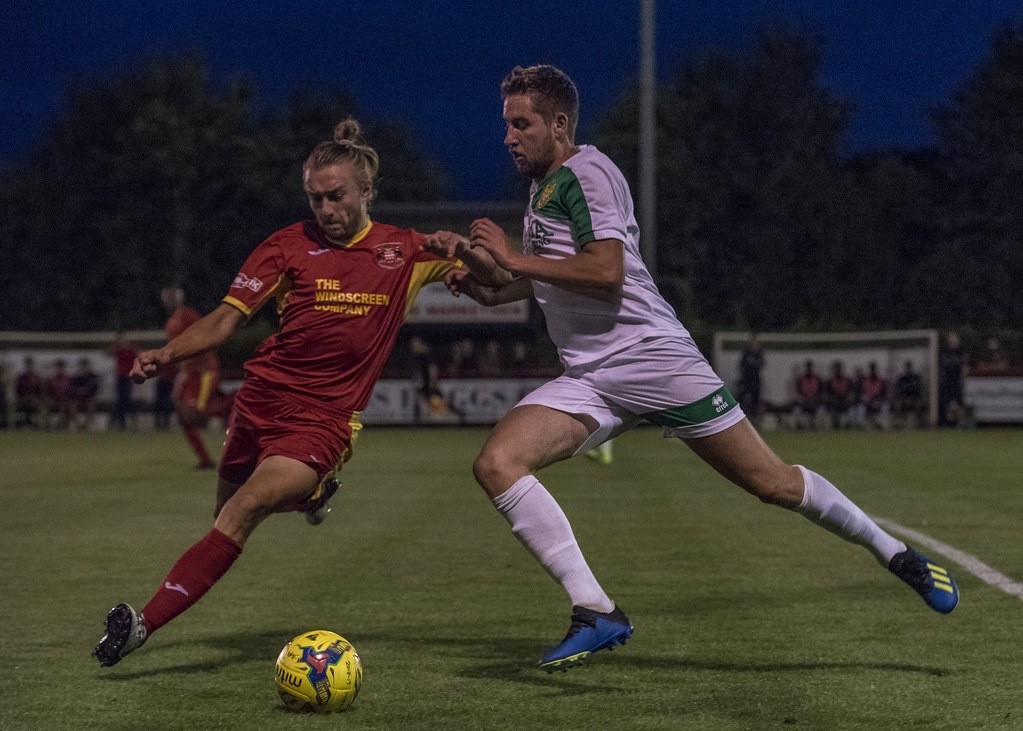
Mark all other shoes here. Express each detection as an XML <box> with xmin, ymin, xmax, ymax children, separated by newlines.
<box><xmin>193</xmin><ymin>459</ymin><xmax>219</xmax><ymax>472</ymax></box>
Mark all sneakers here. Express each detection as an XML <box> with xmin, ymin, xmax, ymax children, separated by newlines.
<box><xmin>90</xmin><ymin>602</ymin><xmax>147</xmax><ymax>668</ymax></box>
<box><xmin>888</xmin><ymin>544</ymin><xmax>960</xmax><ymax>614</ymax></box>
<box><xmin>306</xmin><ymin>478</ymin><xmax>341</xmax><ymax>526</ymax></box>
<box><xmin>535</xmin><ymin>601</ymin><xmax>635</xmax><ymax>675</ymax></box>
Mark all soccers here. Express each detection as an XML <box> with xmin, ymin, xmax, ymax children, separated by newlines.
<box><xmin>276</xmin><ymin>630</ymin><xmax>362</xmax><ymax>710</ymax></box>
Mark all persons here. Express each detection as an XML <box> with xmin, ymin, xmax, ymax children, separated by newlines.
<box><xmin>71</xmin><ymin>356</ymin><xmax>104</xmax><ymax>435</ymax></box>
<box><xmin>14</xmin><ymin>354</ymin><xmax>51</xmax><ymax>430</ymax></box>
<box><xmin>886</xmin><ymin>357</ymin><xmax>931</xmax><ymax>432</ymax></box>
<box><xmin>411</xmin><ymin>336</ymin><xmax>468</xmax><ymax>429</ymax></box>
<box><xmin>92</xmin><ymin>121</ymin><xmax>511</xmax><ymax>668</ymax></box>
<box><xmin>45</xmin><ymin>358</ymin><xmax>73</xmax><ymax>432</ymax></box>
<box><xmin>153</xmin><ymin>285</ymin><xmax>237</xmax><ymax>471</ymax></box>
<box><xmin>822</xmin><ymin>359</ymin><xmax>859</xmax><ymax>431</ymax></box>
<box><xmin>859</xmin><ymin>361</ymin><xmax>887</xmax><ymax>431</ymax></box>
<box><xmin>445</xmin><ymin>64</ymin><xmax>959</xmax><ymax>670</ymax></box>
<box><xmin>943</xmin><ymin>326</ymin><xmax>1010</xmax><ymax>429</ymax></box>
<box><xmin>735</xmin><ymin>338</ymin><xmax>768</xmax><ymax>431</ymax></box>
<box><xmin>791</xmin><ymin>360</ymin><xmax>822</xmax><ymax>432</ymax></box>
<box><xmin>105</xmin><ymin>328</ymin><xmax>140</xmax><ymax>434</ymax></box>
